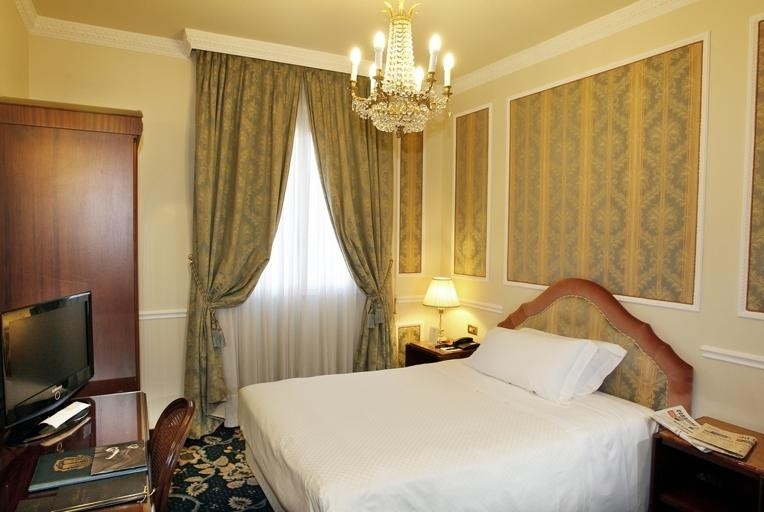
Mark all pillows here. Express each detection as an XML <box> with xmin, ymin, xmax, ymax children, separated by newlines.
<box><xmin>518</xmin><ymin>328</ymin><xmax>628</xmax><ymax>396</ymax></box>
<box><xmin>464</xmin><ymin>326</ymin><xmax>594</xmax><ymax>406</ymax></box>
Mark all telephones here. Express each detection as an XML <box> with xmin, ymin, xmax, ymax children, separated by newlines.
<box><xmin>451</xmin><ymin>337</ymin><xmax>480</xmax><ymax>351</ymax></box>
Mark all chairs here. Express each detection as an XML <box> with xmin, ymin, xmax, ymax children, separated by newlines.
<box><xmin>151</xmin><ymin>397</ymin><xmax>195</xmax><ymax>512</ymax></box>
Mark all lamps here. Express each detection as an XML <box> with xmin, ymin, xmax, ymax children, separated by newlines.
<box><xmin>347</xmin><ymin>0</ymin><xmax>455</xmax><ymax>140</ymax></box>
<box><xmin>422</xmin><ymin>277</ymin><xmax>461</xmax><ymax>343</ymax></box>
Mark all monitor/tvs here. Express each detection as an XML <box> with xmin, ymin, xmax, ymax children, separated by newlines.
<box><xmin>0</xmin><ymin>291</ymin><xmax>94</xmax><ymax>444</ymax></box>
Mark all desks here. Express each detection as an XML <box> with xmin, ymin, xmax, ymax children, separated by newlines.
<box><xmin>0</xmin><ymin>391</ymin><xmax>155</xmax><ymax>512</ymax></box>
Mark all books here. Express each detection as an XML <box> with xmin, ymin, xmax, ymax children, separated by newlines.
<box><xmin>14</xmin><ymin>437</ymin><xmax>150</xmax><ymax>512</ymax></box>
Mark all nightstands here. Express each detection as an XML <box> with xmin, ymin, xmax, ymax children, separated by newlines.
<box><xmin>649</xmin><ymin>414</ymin><xmax>763</xmax><ymax>512</ymax></box>
<box><xmin>405</xmin><ymin>338</ymin><xmax>480</xmax><ymax>367</ymax></box>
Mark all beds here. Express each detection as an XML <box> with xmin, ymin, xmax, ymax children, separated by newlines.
<box><xmin>237</xmin><ymin>279</ymin><xmax>694</xmax><ymax>512</ymax></box>
